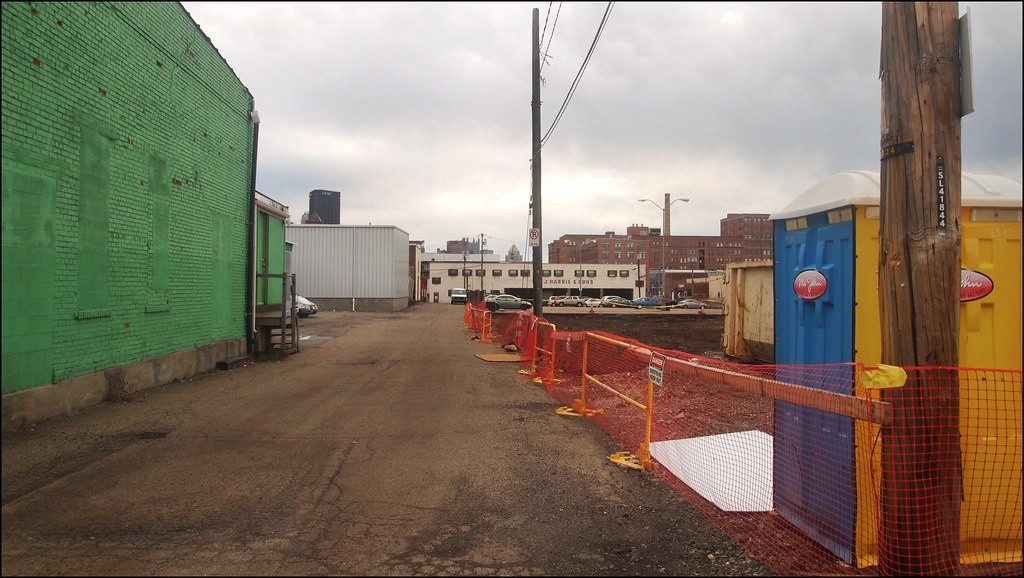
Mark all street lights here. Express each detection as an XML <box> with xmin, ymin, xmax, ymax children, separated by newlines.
<box><xmin>638</xmin><ymin>198</ymin><xmax>690</xmax><ymax>298</ymax></box>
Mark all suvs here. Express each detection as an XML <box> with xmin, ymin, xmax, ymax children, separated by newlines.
<box><xmin>450</xmin><ymin>287</ymin><xmax>468</xmax><ymax>304</ymax></box>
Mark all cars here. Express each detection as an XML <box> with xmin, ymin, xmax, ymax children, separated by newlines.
<box><xmin>484</xmin><ymin>294</ymin><xmax>532</xmax><ymax>311</ymax></box>
<box><xmin>629</xmin><ymin>297</ymin><xmax>663</xmax><ymax>310</ymax></box>
<box><xmin>547</xmin><ymin>295</ymin><xmax>565</xmax><ymax>307</ymax></box>
<box><xmin>581</xmin><ymin>297</ymin><xmax>602</xmax><ymax>307</ymax></box>
<box><xmin>291</xmin><ymin>295</ymin><xmax>318</xmax><ymax>318</ymax></box>
<box><xmin>554</xmin><ymin>295</ymin><xmax>586</xmax><ymax>307</ymax></box>
<box><xmin>677</xmin><ymin>299</ymin><xmax>707</xmax><ymax>309</ymax></box>
<box><xmin>601</xmin><ymin>296</ymin><xmax>632</xmax><ymax>308</ymax></box>
